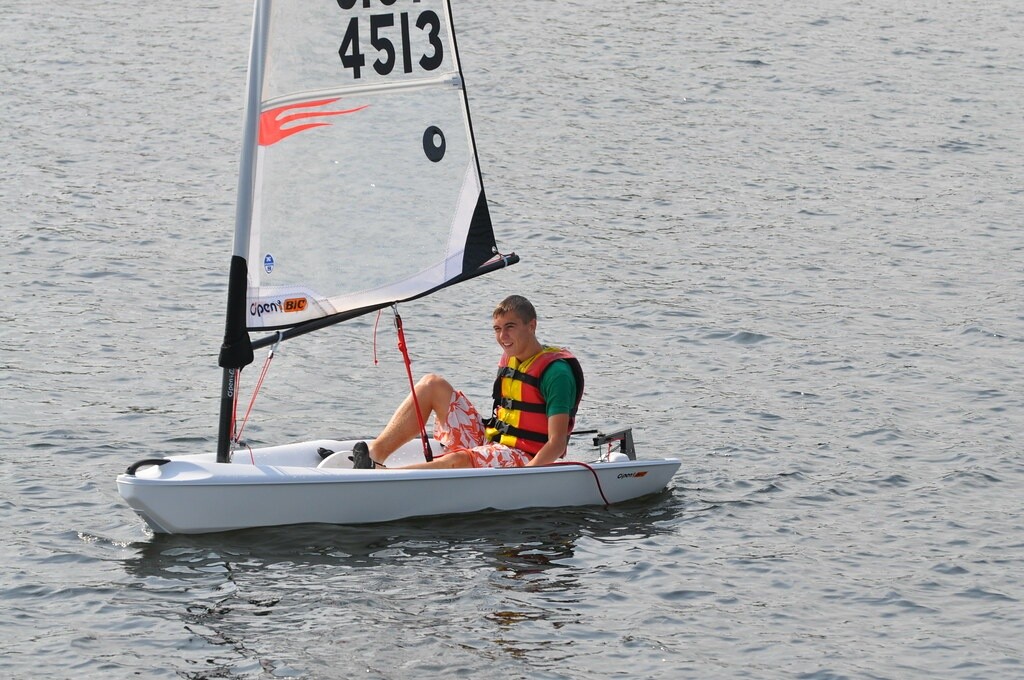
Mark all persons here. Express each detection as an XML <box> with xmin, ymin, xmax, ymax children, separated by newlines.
<box><xmin>316</xmin><ymin>295</ymin><xmax>584</xmax><ymax>469</ymax></box>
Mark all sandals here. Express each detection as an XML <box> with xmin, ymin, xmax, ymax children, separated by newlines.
<box><xmin>317</xmin><ymin>447</ymin><xmax>335</xmax><ymax>458</ymax></box>
<box><xmin>351</xmin><ymin>441</ymin><xmax>376</xmax><ymax>469</ymax></box>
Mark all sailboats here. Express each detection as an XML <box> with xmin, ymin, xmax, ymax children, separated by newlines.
<box><xmin>114</xmin><ymin>0</ymin><xmax>684</xmax><ymax>537</ymax></box>
